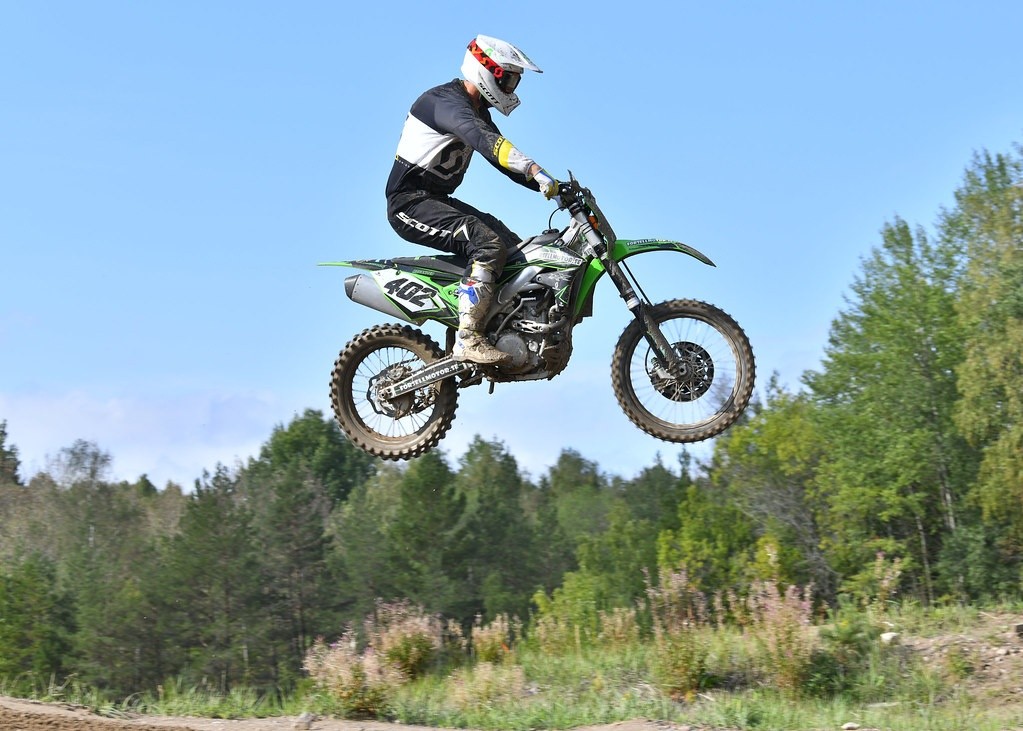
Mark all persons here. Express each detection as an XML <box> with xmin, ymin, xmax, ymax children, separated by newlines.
<box><xmin>383</xmin><ymin>35</ymin><xmax>562</xmax><ymax>366</ymax></box>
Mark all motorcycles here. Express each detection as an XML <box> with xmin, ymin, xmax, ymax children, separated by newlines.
<box><xmin>318</xmin><ymin>174</ymin><xmax>757</xmax><ymax>463</ymax></box>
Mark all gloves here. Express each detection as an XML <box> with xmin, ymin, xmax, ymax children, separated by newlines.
<box><xmin>532</xmin><ymin>167</ymin><xmax>559</xmax><ymax>200</ymax></box>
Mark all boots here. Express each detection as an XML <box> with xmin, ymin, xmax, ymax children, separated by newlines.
<box><xmin>453</xmin><ymin>277</ymin><xmax>513</xmax><ymax>367</ymax></box>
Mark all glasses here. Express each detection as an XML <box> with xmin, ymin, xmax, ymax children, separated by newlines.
<box><xmin>496</xmin><ymin>70</ymin><xmax>521</xmax><ymax>94</ymax></box>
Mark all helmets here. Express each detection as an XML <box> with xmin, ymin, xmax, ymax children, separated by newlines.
<box><xmin>460</xmin><ymin>33</ymin><xmax>544</xmax><ymax>117</ymax></box>
